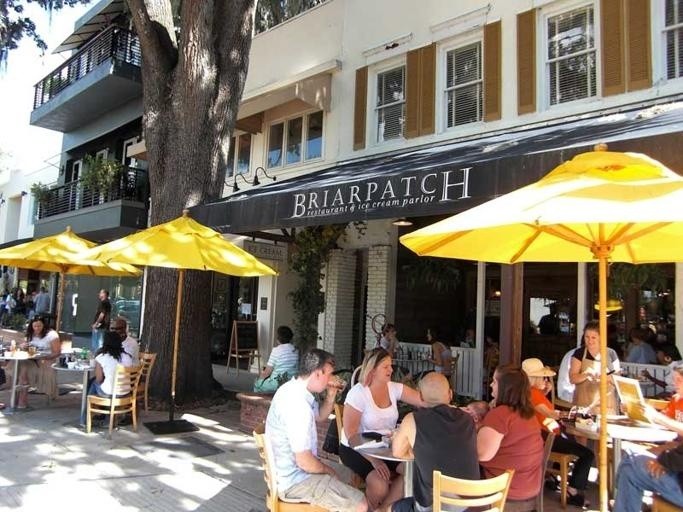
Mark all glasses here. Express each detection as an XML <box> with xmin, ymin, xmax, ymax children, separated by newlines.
<box><xmin>110</xmin><ymin>327</ymin><xmax>124</xmax><ymax>331</ymax></box>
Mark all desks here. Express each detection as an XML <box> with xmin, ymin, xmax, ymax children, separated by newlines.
<box><xmin>51</xmin><ymin>359</ymin><xmax>95</xmax><ymax>428</ymax></box>
<box><xmin>0</xmin><ymin>350</ymin><xmax>52</xmax><ymax>415</ymax></box>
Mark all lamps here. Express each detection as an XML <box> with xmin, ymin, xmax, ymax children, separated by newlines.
<box><xmin>252</xmin><ymin>167</ymin><xmax>277</xmax><ymax>186</ymax></box>
<box><xmin>232</xmin><ymin>173</ymin><xmax>253</xmax><ymax>192</ymax></box>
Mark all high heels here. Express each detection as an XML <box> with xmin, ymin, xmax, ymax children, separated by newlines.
<box><xmin>561</xmin><ymin>490</ymin><xmax>591</xmax><ymax>506</ymax></box>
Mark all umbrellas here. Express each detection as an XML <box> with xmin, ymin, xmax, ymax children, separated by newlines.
<box><xmin>398</xmin><ymin>144</ymin><xmax>683</xmax><ymax>512</ymax></box>
<box><xmin>0</xmin><ymin>226</ymin><xmax>143</xmax><ymax>333</ymax></box>
<box><xmin>74</xmin><ymin>209</ymin><xmax>280</xmax><ymax>422</ymax></box>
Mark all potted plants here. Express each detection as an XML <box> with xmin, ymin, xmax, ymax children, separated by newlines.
<box><xmin>30</xmin><ymin>181</ymin><xmax>55</xmax><ymax>217</ymax></box>
<box><xmin>79</xmin><ymin>152</ymin><xmax>127</xmax><ymax>204</ymax></box>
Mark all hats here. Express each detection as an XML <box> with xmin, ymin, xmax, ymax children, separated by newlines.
<box><xmin>520</xmin><ymin>358</ymin><xmax>557</xmax><ymax>378</ymax></box>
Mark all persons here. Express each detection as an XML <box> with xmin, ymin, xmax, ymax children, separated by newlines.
<box><xmin>0</xmin><ymin>285</ymin><xmax>50</xmax><ymax>315</ymax></box>
<box><xmin>252</xmin><ymin>304</ymin><xmax>683</xmax><ymax>512</ymax></box>
<box><xmin>109</xmin><ymin>319</ymin><xmax>142</xmax><ymax>425</ymax></box>
<box><xmin>0</xmin><ymin>358</ymin><xmax>10</xmax><ymax>411</ymax></box>
<box><xmin>91</xmin><ymin>288</ymin><xmax>110</xmax><ymax>349</ymax></box>
<box><xmin>80</xmin><ymin>331</ymin><xmax>132</xmax><ymax>429</ymax></box>
<box><xmin>15</xmin><ymin>318</ymin><xmax>62</xmax><ymax>406</ymax></box>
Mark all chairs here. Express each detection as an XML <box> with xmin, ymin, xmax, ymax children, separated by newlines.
<box><xmin>86</xmin><ymin>364</ymin><xmax>145</xmax><ymax>434</ymax></box>
<box><xmin>136</xmin><ymin>352</ymin><xmax>158</xmax><ymax>417</ymax></box>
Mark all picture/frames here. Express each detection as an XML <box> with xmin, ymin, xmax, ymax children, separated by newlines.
<box><xmin>214</xmin><ymin>293</ymin><xmax>226</xmax><ymax>304</ymax></box>
<box><xmin>214</xmin><ymin>278</ymin><xmax>226</xmax><ymax>291</ymax></box>
<box><xmin>472</xmin><ymin>275</ymin><xmax>501</xmax><ymax>314</ymax></box>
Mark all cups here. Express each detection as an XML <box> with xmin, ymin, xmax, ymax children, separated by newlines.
<box><xmin>620</xmin><ymin>365</ymin><xmax>651</xmax><ymax>382</ymax></box>
<box><xmin>56</xmin><ymin>346</ymin><xmax>95</xmax><ymax>369</ymax></box>
<box><xmin>381</xmin><ymin>435</ymin><xmax>392</xmax><ymax>449</ymax></box>
<box><xmin>407</xmin><ymin>350</ymin><xmax>428</xmax><ymax>359</ymax></box>
<box><xmin>328</xmin><ymin>376</ymin><xmax>348</xmax><ymax>391</ymax></box>
<box><xmin>0</xmin><ymin>336</ymin><xmax>37</xmax><ymax>360</ymax></box>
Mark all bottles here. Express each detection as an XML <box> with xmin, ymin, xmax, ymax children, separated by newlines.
<box><xmin>595</xmin><ymin>414</ymin><xmax>602</xmax><ymax>432</ymax></box>
<box><xmin>585</xmin><ymin>410</ymin><xmax>595</xmax><ymax>421</ymax></box>
<box><xmin>573</xmin><ymin>409</ymin><xmax>584</xmax><ymax>422</ymax></box>
